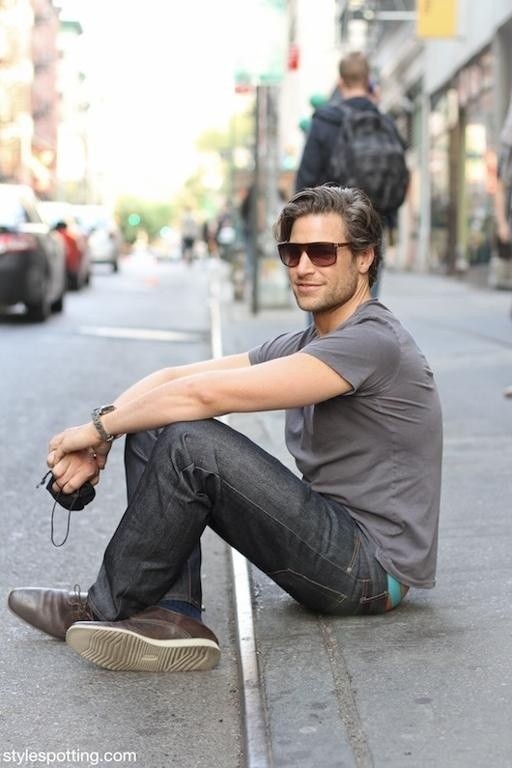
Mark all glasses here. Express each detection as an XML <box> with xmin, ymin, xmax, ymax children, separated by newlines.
<box><xmin>276</xmin><ymin>240</ymin><xmax>369</xmax><ymax>268</ymax></box>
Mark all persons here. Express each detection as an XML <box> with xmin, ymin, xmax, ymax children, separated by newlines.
<box><xmin>292</xmin><ymin>50</ymin><xmax>412</xmax><ymax>316</ymax></box>
<box><xmin>1</xmin><ymin>177</ymin><xmax>448</xmax><ymax>676</ymax></box>
<box><xmin>486</xmin><ymin>103</ymin><xmax>512</xmax><ymax>401</ymax></box>
<box><xmin>177</xmin><ymin>206</ymin><xmax>199</xmax><ymax>261</ymax></box>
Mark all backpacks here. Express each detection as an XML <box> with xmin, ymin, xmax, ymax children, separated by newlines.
<box><xmin>332</xmin><ymin>98</ymin><xmax>410</xmax><ymax>215</ymax></box>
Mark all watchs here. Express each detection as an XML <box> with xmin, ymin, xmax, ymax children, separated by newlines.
<box><xmin>91</xmin><ymin>404</ymin><xmax>126</xmax><ymax>442</ymax></box>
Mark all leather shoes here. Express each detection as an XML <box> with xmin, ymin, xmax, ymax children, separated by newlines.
<box><xmin>65</xmin><ymin>606</ymin><xmax>221</xmax><ymax>672</ymax></box>
<box><xmin>9</xmin><ymin>586</ymin><xmax>97</xmax><ymax>641</ymax></box>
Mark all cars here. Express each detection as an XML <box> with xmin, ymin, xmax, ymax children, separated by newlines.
<box><xmin>0</xmin><ymin>180</ymin><xmax>122</xmax><ymax>322</ymax></box>
